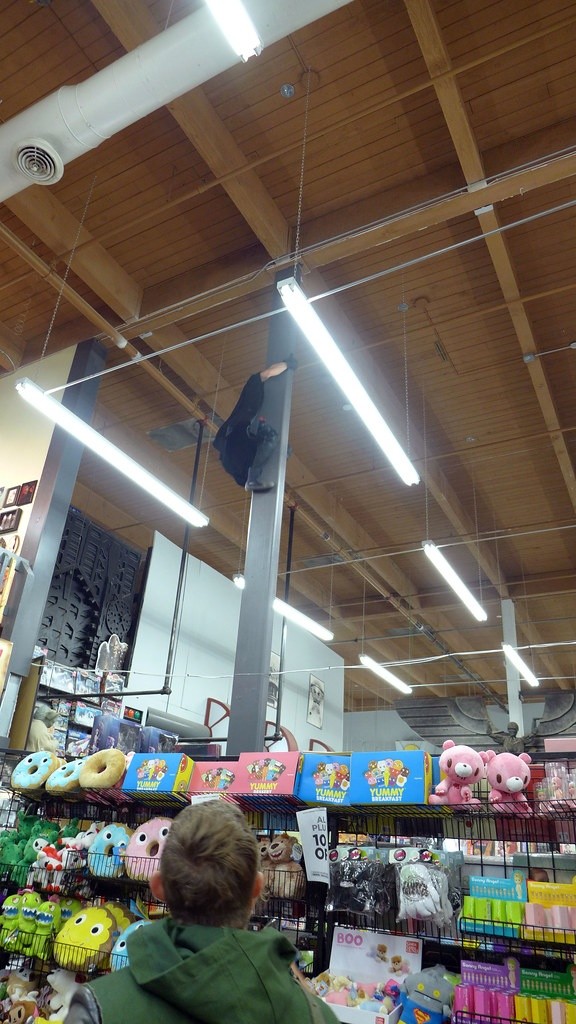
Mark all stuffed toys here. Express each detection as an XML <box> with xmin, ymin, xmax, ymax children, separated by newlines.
<box><xmin>0</xmin><ymin>967</ymin><xmax>86</xmax><ymax>1024</ymax></box>
<box><xmin>88</xmin><ymin>817</ymin><xmax>174</xmax><ymax>882</ymax></box>
<box><xmin>10</xmin><ymin>749</ymin><xmax>126</xmax><ymax>795</ymax></box>
<box><xmin>256</xmin><ymin>833</ymin><xmax>306</xmax><ymax>900</ymax></box>
<box><xmin>303</xmin><ymin>972</ymin><xmax>401</xmax><ymax>1015</ymax></box>
<box><xmin>0</xmin><ymin>887</ymin><xmax>83</xmax><ymax>961</ymax></box>
<box><xmin>396</xmin><ymin>964</ymin><xmax>453</xmax><ymax>1024</ymax></box>
<box><xmin>0</xmin><ymin>811</ymin><xmax>98</xmax><ymax>898</ymax></box>
<box><xmin>53</xmin><ymin>900</ymin><xmax>153</xmax><ymax>973</ymax></box>
<box><xmin>428</xmin><ymin>740</ymin><xmax>489</xmax><ymax>812</ymax></box>
<box><xmin>486</xmin><ymin>750</ymin><xmax>533</xmax><ymax>818</ymax></box>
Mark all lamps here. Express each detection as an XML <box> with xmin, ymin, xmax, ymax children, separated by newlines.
<box><xmin>16</xmin><ymin>61</ymin><xmax>539</xmax><ymax>694</ymax></box>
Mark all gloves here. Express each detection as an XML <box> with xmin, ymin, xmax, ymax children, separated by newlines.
<box><xmin>396</xmin><ymin>863</ymin><xmax>453</xmax><ymax>929</ymax></box>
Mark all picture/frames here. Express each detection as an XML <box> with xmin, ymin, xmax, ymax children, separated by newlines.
<box><xmin>3</xmin><ymin>485</ymin><xmax>21</xmax><ymax>508</ymax></box>
<box><xmin>16</xmin><ymin>480</ymin><xmax>37</xmax><ymax>506</ymax></box>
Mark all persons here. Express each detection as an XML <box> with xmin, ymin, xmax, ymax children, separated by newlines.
<box><xmin>212</xmin><ymin>353</ymin><xmax>298</xmax><ymax>492</ymax></box>
<box><xmin>487</xmin><ymin>722</ymin><xmax>538</xmax><ymax>752</ymax></box>
<box><xmin>536</xmin><ymin>775</ymin><xmax>576</xmax><ymax>816</ymax></box>
<box><xmin>64</xmin><ymin>800</ymin><xmax>339</xmax><ymax>1024</ymax></box>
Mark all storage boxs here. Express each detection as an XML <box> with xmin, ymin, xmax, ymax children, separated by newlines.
<box><xmin>306</xmin><ymin>969</ymin><xmax>403</xmax><ymax>1024</ymax></box>
<box><xmin>120</xmin><ymin>749</ymin><xmax>446</xmax><ymax>808</ymax></box>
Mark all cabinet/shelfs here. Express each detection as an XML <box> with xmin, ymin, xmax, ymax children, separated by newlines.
<box><xmin>0</xmin><ymin>787</ymin><xmax>576</xmax><ymax>1024</ymax></box>
